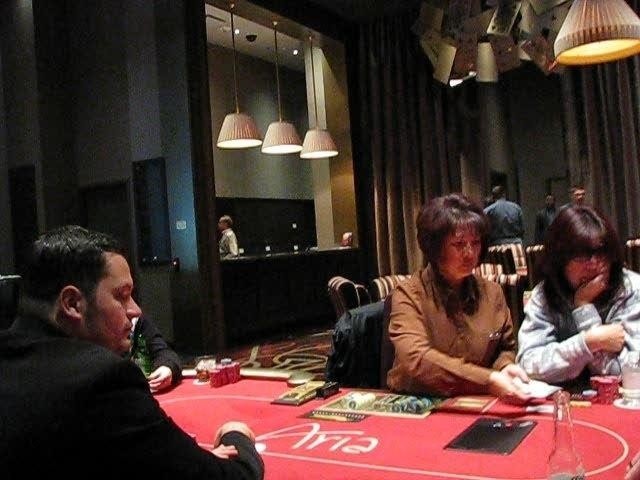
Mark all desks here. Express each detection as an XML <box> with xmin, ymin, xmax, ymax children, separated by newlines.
<box><xmin>144</xmin><ymin>357</ymin><xmax>640</xmax><ymax>480</ymax></box>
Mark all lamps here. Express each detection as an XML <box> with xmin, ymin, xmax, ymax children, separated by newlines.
<box><xmin>411</xmin><ymin>2</ymin><xmax>559</xmax><ymax>89</ymax></box>
<box><xmin>216</xmin><ymin>1</ymin><xmax>340</xmax><ymax>162</ymax></box>
<box><xmin>549</xmin><ymin>1</ymin><xmax>640</xmax><ymax>68</ymax></box>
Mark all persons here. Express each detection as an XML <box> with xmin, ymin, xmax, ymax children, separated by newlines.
<box><xmin>535</xmin><ymin>193</ymin><xmax>559</xmax><ymax>246</ymax></box>
<box><xmin>483</xmin><ymin>186</ymin><xmax>524</xmax><ymax>250</ymax></box>
<box><xmin>341</xmin><ymin>231</ymin><xmax>354</xmax><ymax>247</ymax></box>
<box><xmin>516</xmin><ymin>203</ymin><xmax>640</xmax><ymax>385</ymax></box>
<box><xmin>218</xmin><ymin>215</ymin><xmax>240</xmax><ymax>259</ymax></box>
<box><xmin>120</xmin><ymin>306</ymin><xmax>183</xmax><ymax>395</ymax></box>
<box><xmin>559</xmin><ymin>186</ymin><xmax>588</xmax><ymax>214</ymax></box>
<box><xmin>0</xmin><ymin>225</ymin><xmax>265</xmax><ymax>478</ymax></box>
<box><xmin>379</xmin><ymin>193</ymin><xmax>550</xmax><ymax>407</ymax></box>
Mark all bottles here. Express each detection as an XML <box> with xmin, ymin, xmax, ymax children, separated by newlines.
<box><xmin>546</xmin><ymin>391</ymin><xmax>585</xmax><ymax>480</ymax></box>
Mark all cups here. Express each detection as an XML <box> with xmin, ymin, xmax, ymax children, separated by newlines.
<box><xmin>195</xmin><ymin>354</ymin><xmax>218</xmax><ymax>382</ymax></box>
<box><xmin>621</xmin><ymin>362</ymin><xmax>640</xmax><ymax>389</ymax></box>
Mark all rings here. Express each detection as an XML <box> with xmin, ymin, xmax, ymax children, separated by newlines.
<box><xmin>165</xmin><ymin>381</ymin><xmax>167</xmax><ymax>385</ymax></box>
